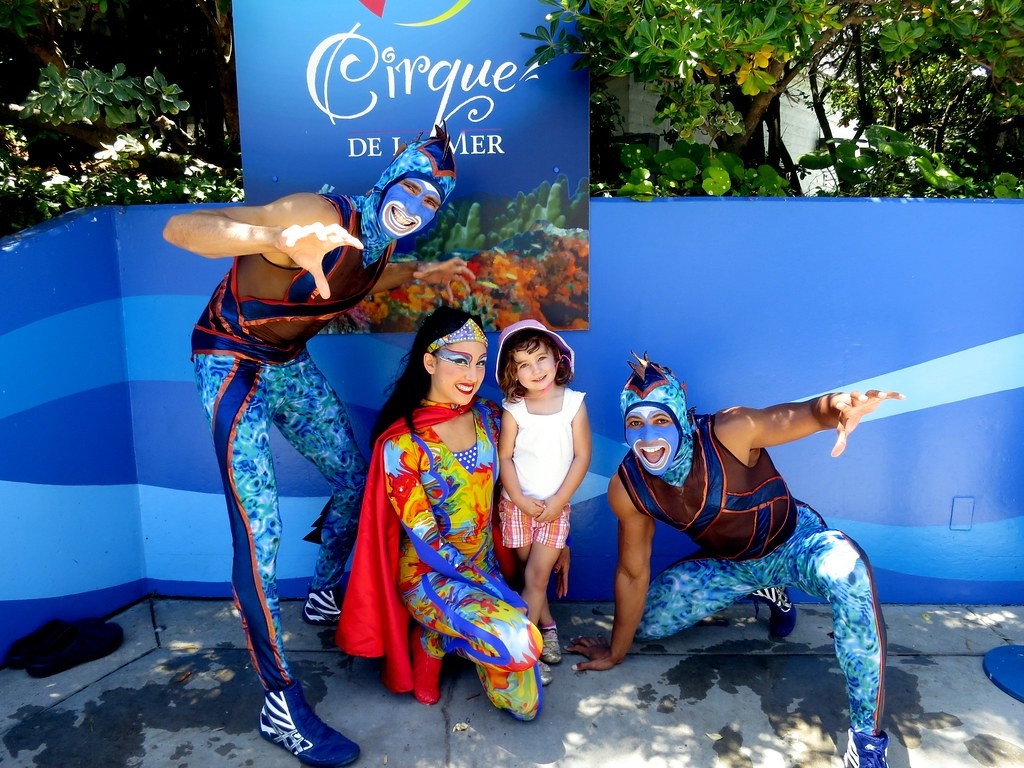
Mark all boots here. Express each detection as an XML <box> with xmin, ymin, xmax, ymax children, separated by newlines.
<box><xmin>259</xmin><ymin>681</ymin><xmax>360</xmax><ymax>766</ymax></box>
<box><xmin>302</xmin><ymin>585</ymin><xmax>344</xmax><ymax>625</ymax></box>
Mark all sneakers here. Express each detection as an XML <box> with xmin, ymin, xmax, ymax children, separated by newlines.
<box><xmin>541</xmin><ymin>632</ymin><xmax>562</xmax><ymax>662</ymax></box>
<box><xmin>845</xmin><ymin>728</ymin><xmax>889</xmax><ymax>768</ymax></box>
<box><xmin>537</xmin><ymin>660</ymin><xmax>552</xmax><ymax>685</ymax></box>
<box><xmin>749</xmin><ymin>584</ymin><xmax>796</xmax><ymax>639</ymax></box>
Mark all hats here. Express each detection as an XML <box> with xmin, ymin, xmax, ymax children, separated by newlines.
<box><xmin>495</xmin><ymin>318</ymin><xmax>575</xmax><ymax>386</ymax></box>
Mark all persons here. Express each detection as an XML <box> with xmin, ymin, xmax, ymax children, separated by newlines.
<box><xmin>495</xmin><ymin>319</ymin><xmax>592</xmax><ymax>686</ymax></box>
<box><xmin>162</xmin><ymin>119</ymin><xmax>476</xmax><ymax>767</ymax></box>
<box><xmin>563</xmin><ymin>351</ymin><xmax>909</xmax><ymax>768</ymax></box>
<box><xmin>370</xmin><ymin>306</ymin><xmax>570</xmax><ymax>722</ymax></box>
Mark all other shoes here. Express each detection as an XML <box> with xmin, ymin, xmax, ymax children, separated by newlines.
<box><xmin>411</xmin><ymin>624</ymin><xmax>442</xmax><ymax>704</ymax></box>
